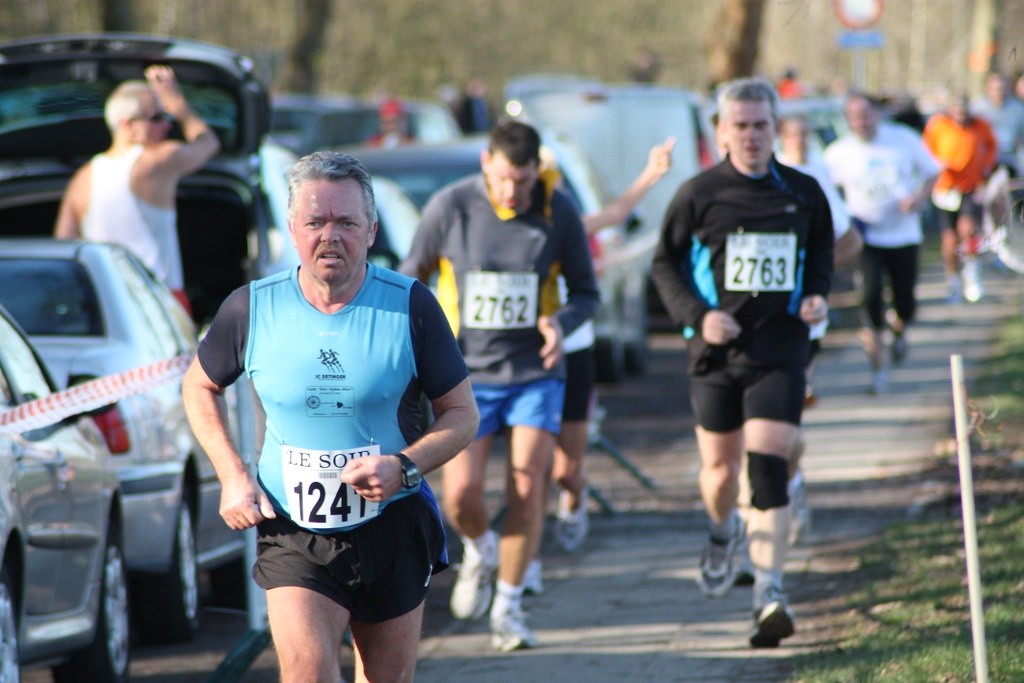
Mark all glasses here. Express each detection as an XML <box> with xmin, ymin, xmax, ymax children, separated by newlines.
<box><xmin>133</xmin><ymin>112</ymin><xmax>163</xmax><ymax>123</ymax></box>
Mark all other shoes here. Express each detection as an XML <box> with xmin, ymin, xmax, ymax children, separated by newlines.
<box><xmin>961</xmin><ymin>260</ymin><xmax>984</xmax><ymax>303</ymax></box>
<box><xmin>788</xmin><ymin>481</ymin><xmax>812</xmax><ymax>543</ymax></box>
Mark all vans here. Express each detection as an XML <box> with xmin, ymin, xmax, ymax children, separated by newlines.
<box><xmin>503</xmin><ymin>75</ymin><xmax>714</xmax><ymax>331</ymax></box>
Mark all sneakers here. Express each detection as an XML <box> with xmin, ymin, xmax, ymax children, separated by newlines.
<box><xmin>554</xmin><ymin>486</ymin><xmax>588</xmax><ymax>550</ymax></box>
<box><xmin>750</xmin><ymin>598</ymin><xmax>793</xmax><ymax>647</ymax></box>
<box><xmin>697</xmin><ymin>517</ymin><xmax>747</xmax><ymax>597</ymax></box>
<box><xmin>523</xmin><ymin>562</ymin><xmax>542</xmax><ymax>595</ymax></box>
<box><xmin>489</xmin><ymin>611</ymin><xmax>537</xmax><ymax>652</ymax></box>
<box><xmin>452</xmin><ymin>535</ymin><xmax>501</xmax><ymax>619</ymax></box>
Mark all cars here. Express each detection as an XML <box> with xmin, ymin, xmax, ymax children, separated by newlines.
<box><xmin>0</xmin><ymin>32</ymin><xmax>626</xmax><ymax>683</ymax></box>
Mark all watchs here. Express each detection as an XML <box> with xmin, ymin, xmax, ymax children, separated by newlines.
<box><xmin>393</xmin><ymin>453</ymin><xmax>420</xmax><ymax>489</ymax></box>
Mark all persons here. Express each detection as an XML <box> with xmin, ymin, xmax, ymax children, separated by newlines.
<box><xmin>56</xmin><ymin>66</ymin><xmax>1024</xmax><ymax>683</ymax></box>
